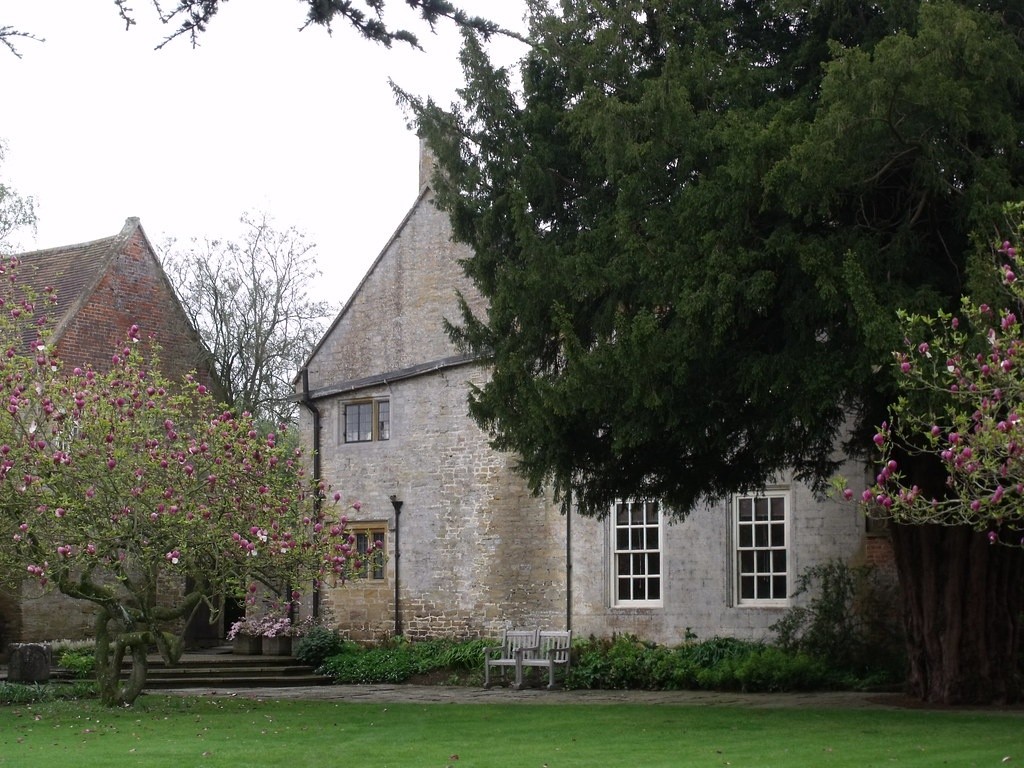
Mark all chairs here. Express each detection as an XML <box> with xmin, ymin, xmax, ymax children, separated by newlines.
<box><xmin>482</xmin><ymin>628</ymin><xmax>537</xmax><ymax>689</ymax></box>
<box><xmin>515</xmin><ymin>630</ymin><xmax>572</xmax><ymax>691</ymax></box>
